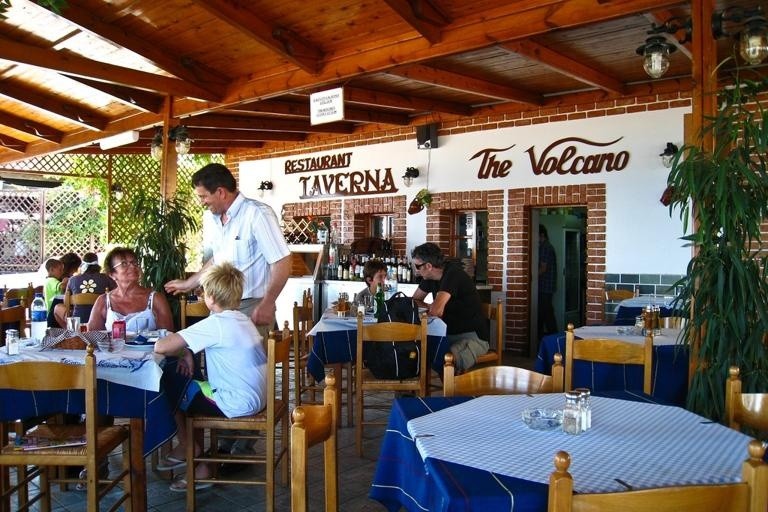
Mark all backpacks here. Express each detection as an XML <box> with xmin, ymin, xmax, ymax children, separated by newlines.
<box><xmin>364</xmin><ymin>290</ymin><xmax>423</xmax><ymax>381</ymax></box>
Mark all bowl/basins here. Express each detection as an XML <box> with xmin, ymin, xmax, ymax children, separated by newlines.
<box><xmin>98</xmin><ymin>339</ymin><xmax>125</xmax><ymax>353</ymax></box>
<box><xmin>21</xmin><ymin>337</ymin><xmax>40</xmax><ymax>346</ymax></box>
<box><xmin>522</xmin><ymin>408</ymin><xmax>562</xmax><ymax>430</ymax></box>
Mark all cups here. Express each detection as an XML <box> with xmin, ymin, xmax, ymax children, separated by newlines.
<box><xmin>136</xmin><ymin>317</ymin><xmax>149</xmax><ymax>336</ymax></box>
<box><xmin>65</xmin><ymin>317</ymin><xmax>80</xmax><ymax>333</ymax></box>
<box><xmin>157</xmin><ymin>329</ymin><xmax>167</xmax><ymax>340</ymax></box>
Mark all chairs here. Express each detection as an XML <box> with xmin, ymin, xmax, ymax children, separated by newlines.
<box><xmin>353</xmin><ymin>311</ymin><xmax>430</xmax><ymax>456</ymax></box>
<box><xmin>443</xmin><ymin>353</ymin><xmax>565</xmax><ymax>400</ymax></box>
<box><xmin>548</xmin><ymin>441</ymin><xmax>768</xmax><ymax>511</ymax></box>
<box><xmin>475</xmin><ymin>299</ymin><xmax>504</xmax><ymax>366</ymax></box>
<box><xmin>605</xmin><ymin>290</ymin><xmax>635</xmax><ymax>302</ymax></box>
<box><xmin>290</xmin><ymin>375</ymin><xmax>338</xmax><ymax>511</ymax></box>
<box><xmin>2</xmin><ymin>285</ymin><xmax>354</xmax><ymax>511</ymax></box>
<box><xmin>564</xmin><ymin>324</ymin><xmax>653</xmax><ymax>397</ymax></box>
<box><xmin>726</xmin><ymin>366</ymin><xmax>768</xmax><ymax>438</ymax></box>
<box><xmin>644</xmin><ymin>310</ymin><xmax>690</xmax><ymax>331</ymax></box>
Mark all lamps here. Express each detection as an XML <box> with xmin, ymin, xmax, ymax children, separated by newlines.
<box><xmin>635</xmin><ymin>10</ymin><xmax>695</xmax><ymax>80</ymax></box>
<box><xmin>167</xmin><ymin>122</ymin><xmax>196</xmax><ymax>158</ymax></box>
<box><xmin>254</xmin><ymin>178</ymin><xmax>274</xmax><ymax>199</ymax></box>
<box><xmin>712</xmin><ymin>1</ymin><xmax>767</xmax><ymax>68</ymax></box>
<box><xmin>146</xmin><ymin>125</ymin><xmax>164</xmax><ymax>162</ymax></box>
<box><xmin>402</xmin><ymin>165</ymin><xmax>420</xmax><ymax>188</ymax></box>
<box><xmin>110</xmin><ymin>182</ymin><xmax>127</xmax><ymax>203</ymax></box>
<box><xmin>656</xmin><ymin>140</ymin><xmax>681</xmax><ymax>169</ymax></box>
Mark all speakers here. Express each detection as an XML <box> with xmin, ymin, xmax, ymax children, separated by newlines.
<box><xmin>417</xmin><ymin>124</ymin><xmax>438</xmax><ymax>148</ymax></box>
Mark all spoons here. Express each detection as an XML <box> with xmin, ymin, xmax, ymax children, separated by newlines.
<box><xmin>107</xmin><ymin>333</ymin><xmax>113</xmax><ymax>352</ymax></box>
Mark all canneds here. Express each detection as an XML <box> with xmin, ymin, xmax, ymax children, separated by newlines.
<box><xmin>113</xmin><ymin>321</ymin><xmax>126</xmax><ymax>339</ymax></box>
<box><xmin>80</xmin><ymin>325</ymin><xmax>87</xmax><ymax>333</ymax></box>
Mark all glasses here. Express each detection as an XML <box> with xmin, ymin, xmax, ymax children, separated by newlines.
<box><xmin>109</xmin><ymin>260</ymin><xmax>140</xmax><ymax>269</ymax></box>
<box><xmin>414</xmin><ymin>263</ymin><xmax>431</xmax><ymax>268</ymax></box>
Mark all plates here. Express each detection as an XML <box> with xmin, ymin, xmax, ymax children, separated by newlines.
<box><xmin>126</xmin><ymin>342</ymin><xmax>156</xmax><ymax>347</ymax></box>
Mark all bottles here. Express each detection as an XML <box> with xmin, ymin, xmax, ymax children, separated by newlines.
<box><xmin>562</xmin><ymin>388</ymin><xmax>591</xmax><ymax>436</ymax></box>
<box><xmin>30</xmin><ymin>293</ymin><xmax>48</xmax><ymax>343</ymax></box>
<box><xmin>4</xmin><ymin>329</ymin><xmax>19</xmax><ymax>355</ymax></box>
<box><xmin>336</xmin><ymin>282</ymin><xmax>385</xmax><ymax>318</ymax></box>
<box><xmin>635</xmin><ymin>304</ymin><xmax>661</xmax><ymax>337</ymax></box>
<box><xmin>134</xmin><ymin>334</ymin><xmax>147</xmax><ymax>344</ymax></box>
<box><xmin>316</xmin><ymin>221</ymin><xmax>413</xmax><ymax>284</ymax></box>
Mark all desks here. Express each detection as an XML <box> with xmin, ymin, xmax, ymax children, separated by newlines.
<box><xmin>367</xmin><ymin>395</ymin><xmax>755</xmax><ymax>511</ymax></box>
<box><xmin>308</xmin><ymin>300</ymin><xmax>447</xmax><ymax>383</ymax></box>
<box><xmin>535</xmin><ymin>327</ymin><xmax>691</xmax><ymax>406</ymax></box>
<box><xmin>615</xmin><ymin>293</ymin><xmax>692</xmax><ymax>324</ymax></box>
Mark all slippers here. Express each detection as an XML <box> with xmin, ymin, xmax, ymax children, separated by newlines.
<box><xmin>168</xmin><ymin>475</ymin><xmax>214</xmax><ymax>493</ymax></box>
<box><xmin>154</xmin><ymin>450</ymin><xmax>206</xmax><ymax>470</ymax></box>
